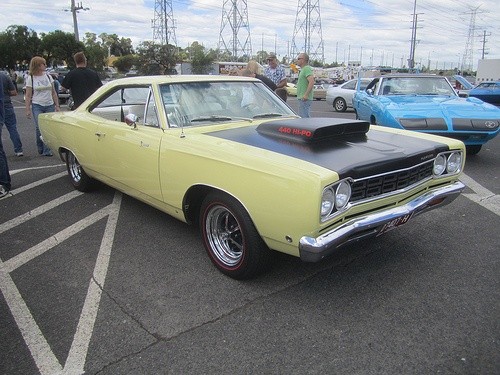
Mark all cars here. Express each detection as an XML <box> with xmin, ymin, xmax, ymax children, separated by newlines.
<box><xmin>48</xmin><ymin>65</ymin><xmax>71</xmax><ymax>100</ymax></box>
<box><xmin>104</xmin><ymin>67</ymin><xmax>117</xmax><ymax>78</ymax></box>
<box><xmin>36</xmin><ymin>75</ymin><xmax>468</xmax><ymax>278</ymax></box>
<box><xmin>283</xmin><ymin>73</ymin><xmax>339</xmax><ymax>101</ymax></box>
<box><xmin>353</xmin><ymin>72</ymin><xmax>500</xmax><ymax>157</ymax></box>
<box><xmin>453</xmin><ymin>75</ymin><xmax>500</xmax><ymax>108</ymax></box>
<box><xmin>325</xmin><ymin>78</ymin><xmax>401</xmax><ymax>112</ymax></box>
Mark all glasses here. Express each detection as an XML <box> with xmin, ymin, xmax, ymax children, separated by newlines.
<box><xmin>297</xmin><ymin>58</ymin><xmax>304</xmax><ymax>60</ymax></box>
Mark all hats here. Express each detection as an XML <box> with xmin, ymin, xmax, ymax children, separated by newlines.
<box><xmin>266</xmin><ymin>55</ymin><xmax>277</xmax><ymax>60</ymax></box>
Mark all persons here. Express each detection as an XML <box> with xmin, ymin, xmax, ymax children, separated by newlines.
<box><xmin>10</xmin><ymin>69</ymin><xmax>18</xmax><ymax>91</ymax></box>
<box><xmin>25</xmin><ymin>56</ymin><xmax>61</xmax><ymax>156</ymax></box>
<box><xmin>296</xmin><ymin>53</ymin><xmax>315</xmax><ymax>118</ymax></box>
<box><xmin>60</xmin><ymin>52</ymin><xmax>103</xmax><ymax>110</ymax></box>
<box><xmin>0</xmin><ymin>72</ymin><xmax>24</xmax><ymax>157</ymax></box>
<box><xmin>0</xmin><ymin>75</ymin><xmax>12</xmax><ymax>198</ymax></box>
<box><xmin>240</xmin><ymin>54</ymin><xmax>288</xmax><ymax>110</ymax></box>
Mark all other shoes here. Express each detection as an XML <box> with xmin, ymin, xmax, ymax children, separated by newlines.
<box><xmin>38</xmin><ymin>150</ymin><xmax>53</xmax><ymax>156</ymax></box>
<box><xmin>15</xmin><ymin>152</ymin><xmax>24</xmax><ymax>156</ymax></box>
<box><xmin>0</xmin><ymin>183</ymin><xmax>10</xmax><ymax>198</ymax></box>
